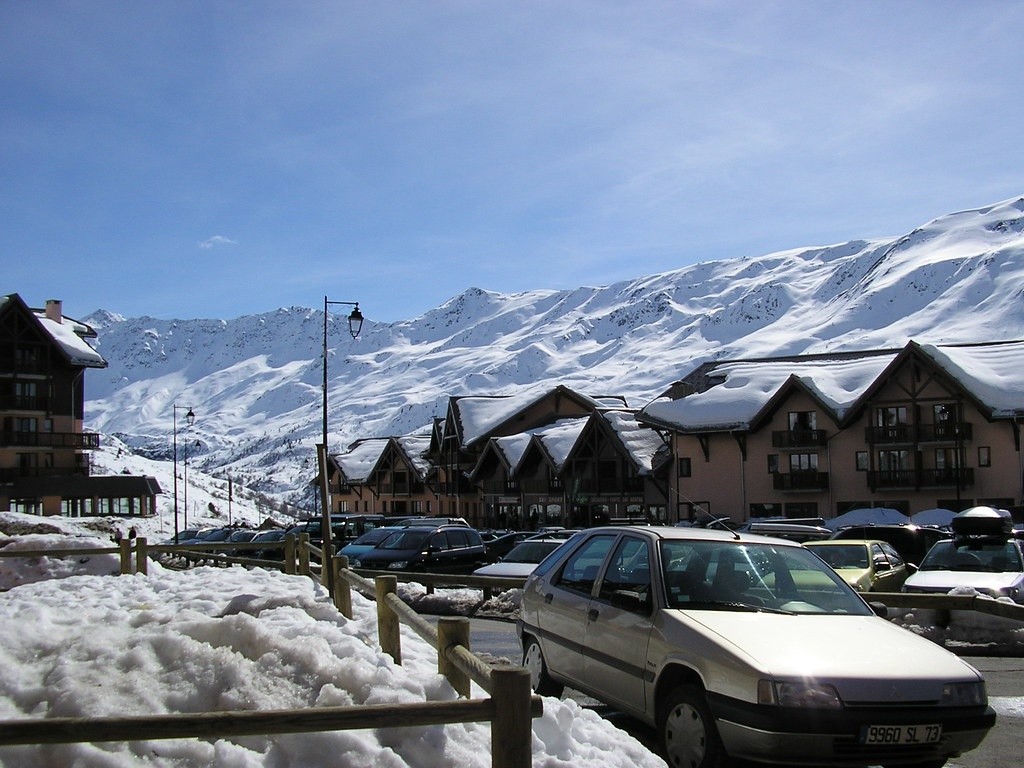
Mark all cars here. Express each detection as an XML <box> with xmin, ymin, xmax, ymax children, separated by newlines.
<box><xmin>899</xmin><ymin>505</ymin><xmax>1024</xmax><ymax>607</ymax></box>
<box><xmin>158</xmin><ymin>513</ymin><xmax>950</xmax><ymax>592</ymax></box>
<box><xmin>515</xmin><ymin>524</ymin><xmax>997</xmax><ymax>768</ymax></box>
<box><xmin>471</xmin><ymin>538</ymin><xmax>569</xmax><ymax>597</ymax></box>
<box><xmin>756</xmin><ymin>538</ymin><xmax>911</xmax><ymax>609</ymax></box>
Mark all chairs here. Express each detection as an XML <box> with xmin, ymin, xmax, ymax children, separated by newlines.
<box><xmin>709</xmin><ymin>569</ymin><xmax>764</xmax><ymax>606</ymax></box>
<box><xmin>583</xmin><ymin>563</ymin><xmax>651</xmax><ymax>588</ymax></box>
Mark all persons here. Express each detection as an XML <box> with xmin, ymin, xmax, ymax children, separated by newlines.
<box><xmin>991</xmin><ymin>547</ymin><xmax>1010</xmax><ymax>569</ymax></box>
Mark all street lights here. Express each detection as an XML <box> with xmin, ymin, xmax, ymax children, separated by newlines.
<box><xmin>173</xmin><ymin>404</ymin><xmax>196</xmax><ymax>544</ymax></box>
<box><xmin>321</xmin><ymin>294</ymin><xmax>365</xmax><ymax>590</ymax></box>
<box><xmin>185</xmin><ymin>437</ymin><xmax>202</xmax><ymax>532</ymax></box>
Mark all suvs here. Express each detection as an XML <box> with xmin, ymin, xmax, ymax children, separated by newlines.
<box><xmin>336</xmin><ymin>522</ymin><xmax>488</xmax><ymax>588</ymax></box>
